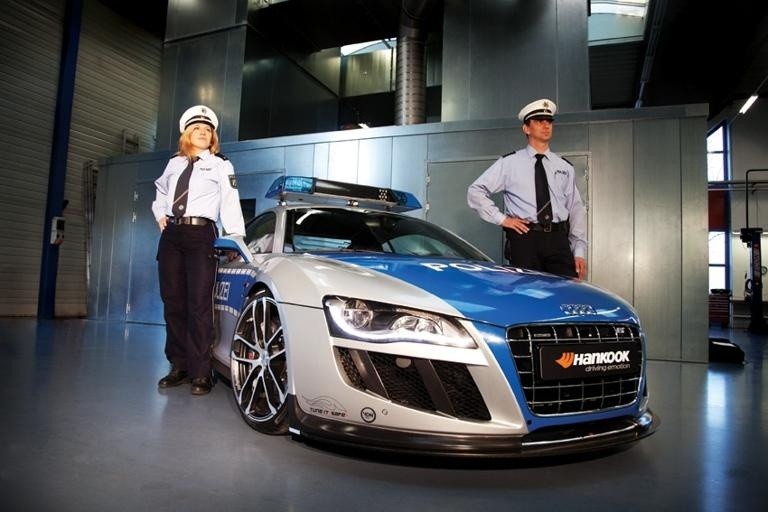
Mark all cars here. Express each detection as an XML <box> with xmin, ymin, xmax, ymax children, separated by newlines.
<box><xmin>210</xmin><ymin>175</ymin><xmax>662</xmax><ymax>463</ymax></box>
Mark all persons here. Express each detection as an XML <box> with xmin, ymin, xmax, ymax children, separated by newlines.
<box><xmin>467</xmin><ymin>98</ymin><xmax>588</xmax><ymax>280</ymax></box>
<box><xmin>152</xmin><ymin>105</ymin><xmax>246</xmax><ymax>395</ymax></box>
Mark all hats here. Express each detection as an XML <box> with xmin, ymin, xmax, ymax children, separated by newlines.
<box><xmin>518</xmin><ymin>98</ymin><xmax>557</xmax><ymax>123</ymax></box>
<box><xmin>179</xmin><ymin>105</ymin><xmax>219</xmax><ymax>133</ymax></box>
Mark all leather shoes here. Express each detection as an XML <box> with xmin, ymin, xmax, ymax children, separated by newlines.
<box><xmin>191</xmin><ymin>377</ymin><xmax>217</xmax><ymax>394</ymax></box>
<box><xmin>159</xmin><ymin>367</ymin><xmax>193</xmax><ymax>388</ymax></box>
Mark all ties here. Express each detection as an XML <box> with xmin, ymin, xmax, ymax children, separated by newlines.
<box><xmin>535</xmin><ymin>154</ymin><xmax>553</xmax><ymax>225</ymax></box>
<box><xmin>171</xmin><ymin>155</ymin><xmax>200</xmax><ymax>219</ymax></box>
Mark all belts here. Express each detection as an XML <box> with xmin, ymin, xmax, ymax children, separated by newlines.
<box><xmin>528</xmin><ymin>222</ymin><xmax>562</xmax><ymax>235</ymax></box>
<box><xmin>166</xmin><ymin>217</ymin><xmax>214</xmax><ymax>232</ymax></box>
